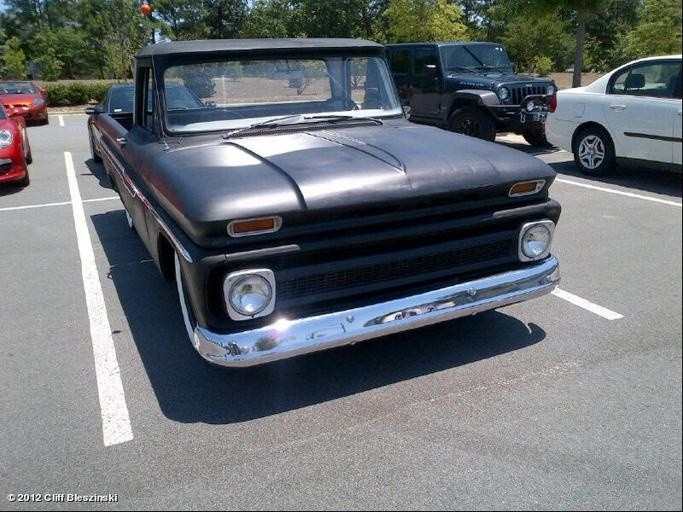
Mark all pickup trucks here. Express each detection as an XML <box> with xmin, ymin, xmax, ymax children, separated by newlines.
<box><xmin>96</xmin><ymin>37</ymin><xmax>561</xmax><ymax>369</ymax></box>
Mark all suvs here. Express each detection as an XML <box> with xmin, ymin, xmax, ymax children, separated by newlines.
<box><xmin>361</xmin><ymin>41</ymin><xmax>558</xmax><ymax>146</ymax></box>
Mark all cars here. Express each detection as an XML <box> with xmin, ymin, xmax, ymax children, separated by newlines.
<box><xmin>0</xmin><ymin>80</ymin><xmax>48</xmax><ymax>124</ymax></box>
<box><xmin>0</xmin><ymin>102</ymin><xmax>31</xmax><ymax>187</ymax></box>
<box><xmin>545</xmin><ymin>54</ymin><xmax>683</xmax><ymax>175</ymax></box>
<box><xmin>84</xmin><ymin>83</ymin><xmax>217</xmax><ymax>161</ymax></box>
